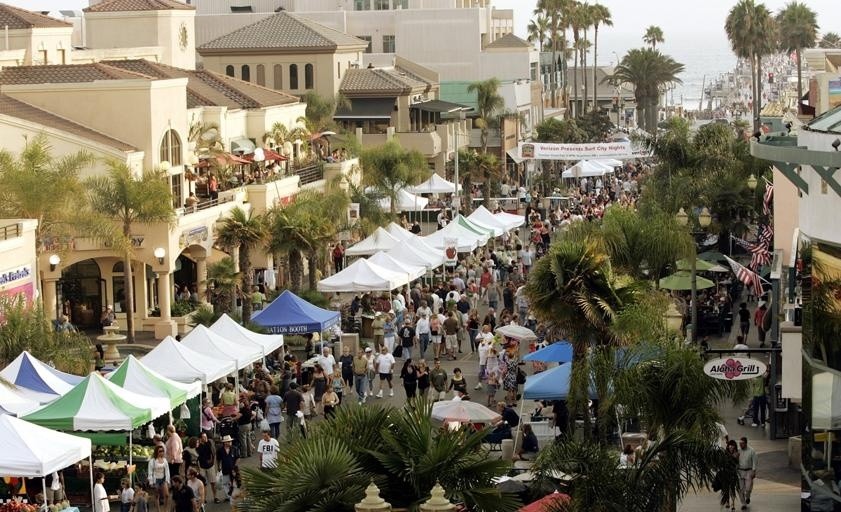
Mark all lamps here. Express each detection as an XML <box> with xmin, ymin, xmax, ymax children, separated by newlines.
<box><xmin>154</xmin><ymin>246</ymin><xmax>167</xmax><ymax>265</ymax></box>
<box><xmin>48</xmin><ymin>254</ymin><xmax>62</xmax><ymax>272</ymax></box>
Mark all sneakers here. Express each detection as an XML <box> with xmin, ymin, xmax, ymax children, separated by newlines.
<box><xmin>342</xmin><ymin>388</ymin><xmax>374</xmax><ymax>406</ymax></box>
<box><xmin>375</xmin><ymin>393</ymin><xmax>383</xmax><ymax>398</ymax></box>
<box><xmin>203</xmin><ymin>497</ymin><xmax>231</xmax><ymax>504</ymax></box>
<box><xmin>418</xmin><ymin>348</ymin><xmax>478</xmax><ymax>364</ymax></box>
<box><xmin>473</xmin><ymin>382</ymin><xmax>519</xmax><ymax>408</ymax></box>
<box><xmin>389</xmin><ymin>392</ymin><xmax>395</xmax><ymax>397</ymax></box>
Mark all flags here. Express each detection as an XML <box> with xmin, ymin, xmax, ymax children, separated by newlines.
<box><xmin>724</xmin><ymin>255</ymin><xmax>767</xmax><ymax>296</ymax></box>
<box><xmin>734</xmin><ymin>177</ymin><xmax>773</xmax><ymax>264</ymax></box>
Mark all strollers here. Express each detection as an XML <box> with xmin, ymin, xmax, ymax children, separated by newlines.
<box><xmin>216</xmin><ymin>417</ymin><xmax>257</xmax><ymax>459</ymax></box>
<box><xmin>534</xmin><ymin>242</ymin><xmax>546</xmax><ymax>259</ymax></box>
<box><xmin>737</xmin><ymin>394</ymin><xmax>763</xmax><ymax>427</ymax></box>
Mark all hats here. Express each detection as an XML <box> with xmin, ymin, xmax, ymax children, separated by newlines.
<box><xmin>220</xmin><ymin>435</ymin><xmax>234</xmax><ymax>443</ymax></box>
<box><xmin>363</xmin><ymin>347</ymin><xmax>373</xmax><ymax>352</ymax></box>
<box><xmin>388</xmin><ymin>309</ymin><xmax>395</xmax><ymax>314</ymax></box>
<box><xmin>107</xmin><ymin>304</ymin><xmax>113</xmax><ymax>310</ymax></box>
<box><xmin>374</xmin><ymin>311</ymin><xmax>382</xmax><ymax>317</ymax></box>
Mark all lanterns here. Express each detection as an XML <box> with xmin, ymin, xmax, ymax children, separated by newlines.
<box><xmin>2</xmin><ymin>289</ymin><xmax>338</xmax><ymax>478</ymax></box>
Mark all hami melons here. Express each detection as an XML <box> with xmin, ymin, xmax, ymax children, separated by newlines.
<box><xmin>48</xmin><ymin>500</ymin><xmax>71</xmax><ymax>511</ymax></box>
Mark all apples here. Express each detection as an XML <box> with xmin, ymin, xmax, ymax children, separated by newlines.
<box><xmin>0</xmin><ymin>501</ymin><xmax>37</xmax><ymax>512</ymax></box>
<box><xmin>446</xmin><ymin>247</ymin><xmax>457</xmax><ymax>259</ymax></box>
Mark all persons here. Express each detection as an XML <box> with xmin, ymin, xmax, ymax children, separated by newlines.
<box><xmin>101</xmin><ymin>304</ymin><xmax>118</xmax><ymax>326</ymax></box>
<box><xmin>339</xmin><ymin>235</ymin><xmax>543</xmax><ymax>457</ymax></box>
<box><xmin>332</xmin><ymin>242</ymin><xmax>345</xmax><ymax>273</ymax></box>
<box><xmin>1</xmin><ymin>341</ymin><xmax>338</xmax><ymax>512</ymax></box>
<box><xmin>501</xmin><ymin>158</ymin><xmax>656</xmax><ymax>259</ymax></box>
<box><xmin>179</xmin><ymin>283</ymin><xmax>265</xmax><ymax>311</ymax></box>
<box><xmin>317</xmin><ymin>144</ymin><xmax>346</xmax><ymax>162</ymax></box>
<box><xmin>684</xmin><ymin>265</ymin><xmax>773</xmax><ymax>347</ymax></box>
<box><xmin>723</xmin><ymin>436</ymin><xmax>758</xmax><ymax>510</ymax></box>
<box><xmin>185</xmin><ymin>159</ymin><xmax>281</xmax><ymax>215</ymax></box>
<box><xmin>400</xmin><ymin>192</ymin><xmax>453</xmax><ymax>235</ymax></box>
<box><xmin>93</xmin><ymin>345</ymin><xmax>106</xmax><ymax>373</ymax></box>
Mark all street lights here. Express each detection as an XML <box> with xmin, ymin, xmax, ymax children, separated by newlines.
<box><xmin>611</xmin><ymin>50</ymin><xmax>620</xmax><ymax>74</ymax></box>
<box><xmin>671</xmin><ymin>205</ymin><xmax>713</xmax><ymax>352</ymax></box>
<box><xmin>747</xmin><ymin>172</ymin><xmax>758</xmax><ymax>234</ymax></box>
<box><xmin>446</xmin><ymin>104</ymin><xmax>476</xmax><ymax>217</ymax></box>
<box><xmin>353</xmin><ymin>477</ymin><xmax>456</xmax><ymax>512</ymax></box>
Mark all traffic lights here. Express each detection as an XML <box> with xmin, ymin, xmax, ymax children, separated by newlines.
<box><xmin>767</xmin><ymin>72</ymin><xmax>773</xmax><ymax>83</ymax></box>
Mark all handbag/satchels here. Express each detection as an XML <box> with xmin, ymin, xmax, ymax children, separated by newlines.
<box><xmin>515</xmin><ymin>366</ymin><xmax>528</xmax><ymax>384</ymax></box>
